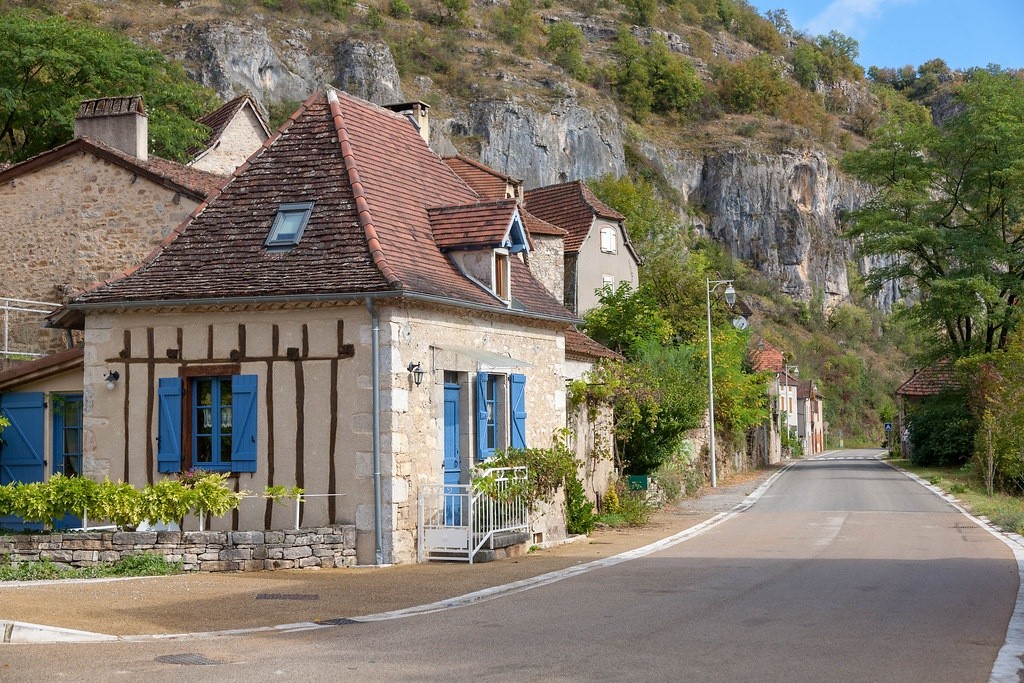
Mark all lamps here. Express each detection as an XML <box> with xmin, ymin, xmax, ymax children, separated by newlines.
<box><xmin>103</xmin><ymin>369</ymin><xmax>120</xmax><ymax>391</ymax></box>
<box><xmin>339</xmin><ymin>344</ymin><xmax>355</xmax><ymax>357</ymax></box>
<box><xmin>287</xmin><ymin>347</ymin><xmax>299</xmax><ymax>360</ymax></box>
<box><xmin>407</xmin><ymin>361</ymin><xmax>428</xmax><ymax>388</ymax></box>
<box><xmin>230</xmin><ymin>349</ymin><xmax>244</xmax><ymax>362</ymax></box>
<box><xmin>165</xmin><ymin>348</ymin><xmax>179</xmax><ymax>359</ymax></box>
<box><xmin>119</xmin><ymin>348</ymin><xmax>129</xmax><ymax>359</ymax></box>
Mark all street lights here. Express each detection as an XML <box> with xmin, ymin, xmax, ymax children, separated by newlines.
<box><xmin>706</xmin><ymin>276</ymin><xmax>736</xmax><ymax>488</ymax></box>
<box><xmin>786</xmin><ymin>364</ymin><xmax>800</xmax><ymax>460</ymax></box>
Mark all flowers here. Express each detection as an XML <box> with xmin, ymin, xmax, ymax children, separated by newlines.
<box><xmin>177</xmin><ymin>465</ymin><xmax>206</xmax><ymax>483</ymax></box>
